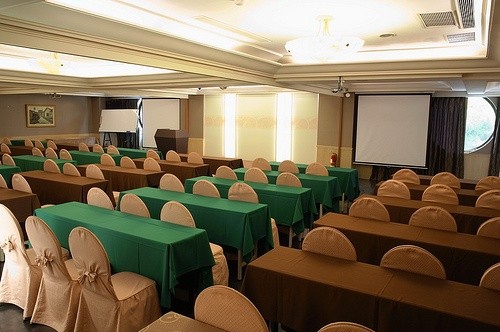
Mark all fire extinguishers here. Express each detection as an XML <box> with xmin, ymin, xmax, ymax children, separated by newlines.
<box><xmin>329</xmin><ymin>153</ymin><xmax>337</xmax><ymax>167</ymax></box>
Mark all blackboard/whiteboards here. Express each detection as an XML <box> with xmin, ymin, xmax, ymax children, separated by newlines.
<box><xmin>98</xmin><ymin>108</ymin><xmax>137</xmax><ymax>134</ymax></box>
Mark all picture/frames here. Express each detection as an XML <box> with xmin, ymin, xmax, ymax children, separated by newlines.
<box><xmin>25</xmin><ymin>104</ymin><xmax>56</xmax><ymax>128</ymax></box>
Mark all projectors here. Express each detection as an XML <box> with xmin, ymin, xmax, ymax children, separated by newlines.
<box><xmin>48</xmin><ymin>94</ymin><xmax>63</xmax><ymax>99</ymax></box>
<box><xmin>332</xmin><ymin>88</ymin><xmax>348</xmax><ymax>94</ymax></box>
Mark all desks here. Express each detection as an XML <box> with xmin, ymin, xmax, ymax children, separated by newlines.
<box><xmin>9</xmin><ymin>144</ymin><xmax>59</xmax><ymax>156</ymax></box>
<box><xmin>312</xmin><ymin>211</ymin><xmax>500</xmax><ymax>285</ymax></box>
<box><xmin>354</xmin><ymin>193</ymin><xmax>499</xmax><ymax>235</ymax></box>
<box><xmin>76</xmin><ymin>163</ymin><xmax>167</xmax><ymax>191</ymax></box>
<box><xmin>241</xmin><ymin>246</ymin><xmax>500</xmax><ymax>332</ymax></box>
<box><xmin>68</xmin><ymin>150</ymin><xmax>124</xmax><ymax>165</ymax></box>
<box><xmin>132</xmin><ymin>156</ymin><xmax>210</xmax><ymax>183</ymax></box>
<box><xmin>269</xmin><ymin>160</ymin><xmax>361</xmax><ymax>213</ymax></box>
<box><xmin>232</xmin><ymin>166</ymin><xmax>339</xmax><ymax>217</ymax></box>
<box><xmin>12</xmin><ymin>170</ymin><xmax>116</xmax><ymax>210</ymax></box>
<box><xmin>0</xmin><ymin>164</ymin><xmax>21</xmax><ymax>188</ymax></box>
<box><xmin>11</xmin><ymin>155</ymin><xmax>78</xmax><ymax>172</ymax></box>
<box><xmin>186</xmin><ymin>176</ymin><xmax>319</xmax><ymax>247</ymax></box>
<box><xmin>117</xmin><ymin>186</ymin><xmax>273</xmax><ymax>280</ymax></box>
<box><xmin>417</xmin><ymin>174</ymin><xmax>478</xmax><ymax>188</ymax></box>
<box><xmin>375</xmin><ymin>181</ymin><xmax>481</xmax><ymax>206</ymax></box>
<box><xmin>33</xmin><ymin>202</ymin><xmax>216</xmax><ymax>315</ymax></box>
<box><xmin>0</xmin><ymin>186</ymin><xmax>41</xmax><ymax>225</ymax></box>
<box><xmin>60</xmin><ymin>142</ymin><xmax>94</xmax><ymax>153</ymax></box>
<box><xmin>177</xmin><ymin>153</ymin><xmax>244</xmax><ymax>175</ymax></box>
<box><xmin>11</xmin><ymin>139</ymin><xmax>48</xmax><ymax>149</ymax></box>
<box><xmin>103</xmin><ymin>146</ymin><xmax>162</xmax><ymax>161</ymax></box>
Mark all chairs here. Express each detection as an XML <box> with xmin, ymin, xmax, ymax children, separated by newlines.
<box><xmin>1</xmin><ymin>142</ymin><xmax>500</xmax><ymax>332</ymax></box>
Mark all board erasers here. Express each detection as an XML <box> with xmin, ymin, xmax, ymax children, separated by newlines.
<box><xmin>127</xmin><ymin>131</ymin><xmax>130</xmax><ymax>132</ymax></box>
